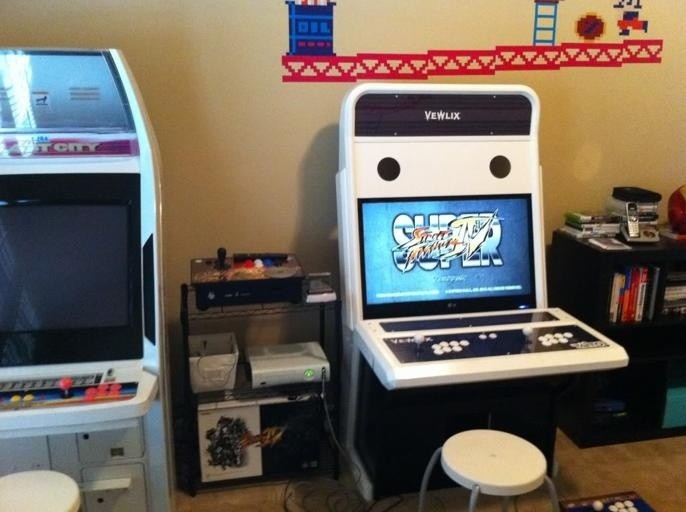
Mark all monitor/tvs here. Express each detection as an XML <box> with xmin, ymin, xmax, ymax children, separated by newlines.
<box><xmin>355</xmin><ymin>192</ymin><xmax>541</xmax><ymax>318</ymax></box>
<box><xmin>0</xmin><ymin>175</ymin><xmax>142</xmax><ymax>365</ymax></box>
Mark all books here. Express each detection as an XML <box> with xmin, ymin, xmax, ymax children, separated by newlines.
<box><xmin>609</xmin><ymin>263</ymin><xmax>686</xmax><ymax>324</ymax></box>
<box><xmin>558</xmin><ymin>211</ymin><xmax>623</xmax><ymax>238</ymax></box>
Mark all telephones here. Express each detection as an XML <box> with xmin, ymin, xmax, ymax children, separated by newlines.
<box><xmin>620</xmin><ymin>202</ymin><xmax>662</xmax><ymax>243</ymax></box>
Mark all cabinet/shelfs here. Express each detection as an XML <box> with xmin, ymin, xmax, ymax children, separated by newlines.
<box><xmin>552</xmin><ymin>230</ymin><xmax>682</xmax><ymax>450</ymax></box>
<box><xmin>173</xmin><ymin>276</ymin><xmax>339</xmax><ymax>494</ymax></box>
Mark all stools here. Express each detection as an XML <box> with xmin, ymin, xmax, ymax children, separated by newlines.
<box><xmin>416</xmin><ymin>426</ymin><xmax>563</xmax><ymax>511</ymax></box>
<box><xmin>1</xmin><ymin>470</ymin><xmax>85</xmax><ymax>512</ymax></box>
